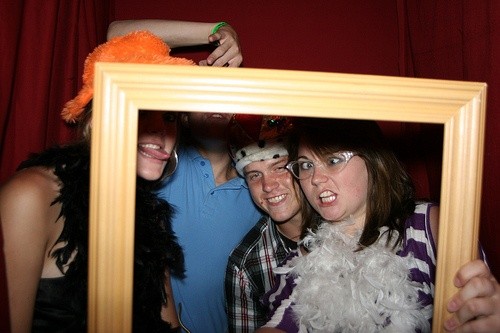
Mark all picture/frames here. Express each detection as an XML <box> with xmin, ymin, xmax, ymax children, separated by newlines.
<box><xmin>87</xmin><ymin>60</ymin><xmax>488</xmax><ymax>332</ymax></box>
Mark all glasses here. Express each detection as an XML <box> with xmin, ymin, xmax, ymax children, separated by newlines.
<box><xmin>285</xmin><ymin>149</ymin><xmax>357</xmax><ymax>180</ymax></box>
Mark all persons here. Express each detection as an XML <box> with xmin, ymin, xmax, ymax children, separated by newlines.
<box><xmin>0</xmin><ymin>30</ymin><xmax>198</xmax><ymax>333</ymax></box>
<box><xmin>255</xmin><ymin>120</ymin><xmax>500</xmax><ymax>333</ymax></box>
<box><xmin>224</xmin><ymin>115</ymin><xmax>324</xmax><ymax>333</ymax></box>
<box><xmin>106</xmin><ymin>19</ymin><xmax>266</xmax><ymax>333</ymax></box>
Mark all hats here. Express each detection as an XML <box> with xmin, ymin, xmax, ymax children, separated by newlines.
<box><xmin>61</xmin><ymin>28</ymin><xmax>199</xmax><ymax>126</ymax></box>
<box><xmin>232</xmin><ymin>138</ymin><xmax>289</xmax><ymax>176</ymax></box>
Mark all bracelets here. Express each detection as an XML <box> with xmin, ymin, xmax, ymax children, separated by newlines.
<box><xmin>210</xmin><ymin>22</ymin><xmax>228</xmax><ymax>35</ymax></box>
